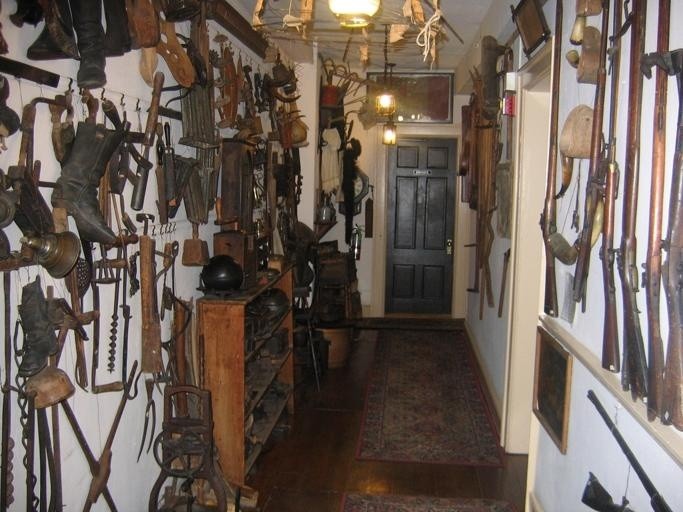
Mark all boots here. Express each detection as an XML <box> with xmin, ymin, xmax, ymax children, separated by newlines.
<box><xmin>13</xmin><ymin>274</ymin><xmax>59</xmax><ymax>383</ymax></box>
<box><xmin>27</xmin><ymin>0</ymin><xmax>163</xmax><ymax>93</ymax></box>
<box><xmin>49</xmin><ymin>119</ymin><xmax>125</xmax><ymax>245</ymax></box>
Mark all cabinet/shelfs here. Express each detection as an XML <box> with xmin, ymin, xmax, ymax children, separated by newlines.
<box><xmin>193</xmin><ymin>260</ymin><xmax>297</xmax><ymax>512</ymax></box>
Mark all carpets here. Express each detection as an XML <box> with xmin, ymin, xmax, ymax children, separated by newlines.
<box><xmin>338</xmin><ymin>492</ymin><xmax>518</xmax><ymax>512</ymax></box>
<box><xmin>354</xmin><ymin>329</ymin><xmax>507</xmax><ymax>468</ymax></box>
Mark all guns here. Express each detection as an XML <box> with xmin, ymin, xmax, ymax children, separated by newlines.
<box><xmin>541</xmin><ymin>0</ymin><xmax>562</xmax><ymax>317</ymax></box>
<box><xmin>601</xmin><ymin>0</ymin><xmax>682</xmax><ymax>432</ymax></box>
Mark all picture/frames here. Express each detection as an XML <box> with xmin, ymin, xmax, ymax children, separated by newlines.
<box><xmin>531</xmin><ymin>325</ymin><xmax>573</xmax><ymax>456</ymax></box>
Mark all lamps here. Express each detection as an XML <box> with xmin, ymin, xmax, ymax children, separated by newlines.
<box><xmin>375</xmin><ymin>22</ymin><xmax>397</xmax><ymax>147</ymax></box>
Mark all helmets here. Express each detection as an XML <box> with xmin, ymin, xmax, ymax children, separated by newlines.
<box><xmin>199</xmin><ymin>254</ymin><xmax>244</xmax><ymax>297</ymax></box>
<box><xmin>26</xmin><ymin>367</ymin><xmax>78</xmax><ymax>413</ymax></box>
